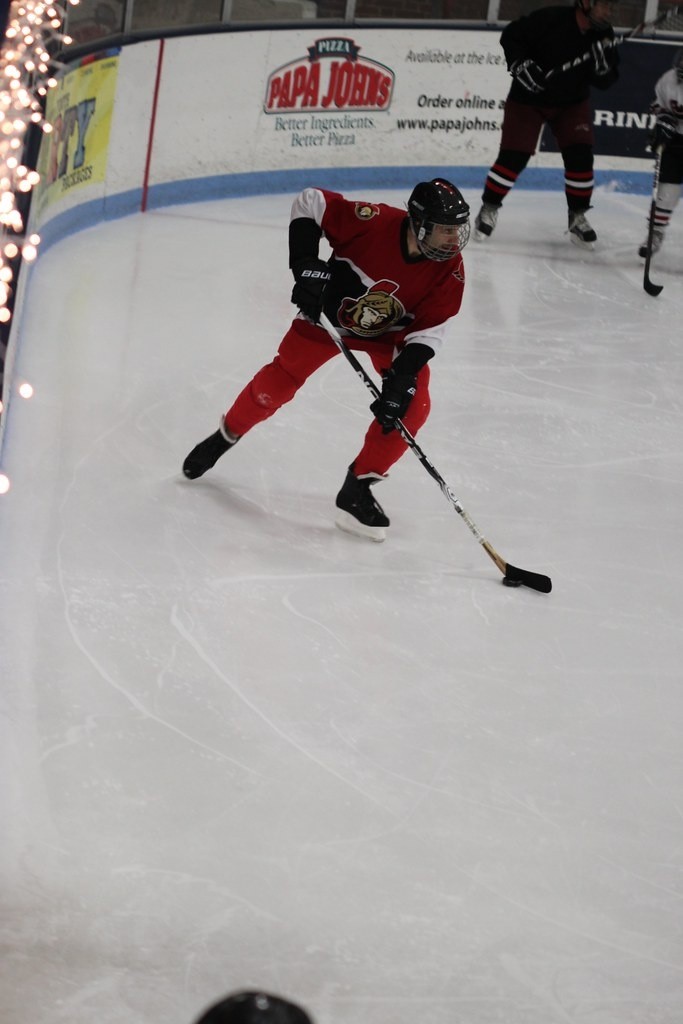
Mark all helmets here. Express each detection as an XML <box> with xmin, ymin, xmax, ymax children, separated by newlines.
<box><xmin>409</xmin><ymin>177</ymin><xmax>472</xmax><ymax>262</ymax></box>
<box><xmin>576</xmin><ymin>0</ymin><xmax>616</xmax><ymax>17</ymax></box>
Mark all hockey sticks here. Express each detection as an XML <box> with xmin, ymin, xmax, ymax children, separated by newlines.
<box><xmin>544</xmin><ymin>14</ymin><xmax>667</xmax><ymax>80</ymax></box>
<box><xmin>643</xmin><ymin>144</ymin><xmax>664</xmax><ymax>296</ymax></box>
<box><xmin>317</xmin><ymin>312</ymin><xmax>553</xmax><ymax>594</ymax></box>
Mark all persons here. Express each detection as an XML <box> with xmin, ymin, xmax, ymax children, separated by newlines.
<box><xmin>471</xmin><ymin>0</ymin><xmax>617</xmax><ymax>251</ymax></box>
<box><xmin>183</xmin><ymin>177</ymin><xmax>472</xmax><ymax>543</ymax></box>
<box><xmin>637</xmin><ymin>52</ymin><xmax>683</xmax><ymax>263</ymax></box>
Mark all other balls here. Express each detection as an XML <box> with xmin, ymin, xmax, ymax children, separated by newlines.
<box><xmin>502</xmin><ymin>576</ymin><xmax>522</xmax><ymax>587</ymax></box>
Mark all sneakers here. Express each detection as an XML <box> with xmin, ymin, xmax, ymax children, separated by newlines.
<box><xmin>565</xmin><ymin>203</ymin><xmax>599</xmax><ymax>250</ymax></box>
<box><xmin>335</xmin><ymin>460</ymin><xmax>392</xmax><ymax>541</ymax></box>
<box><xmin>181</xmin><ymin>414</ymin><xmax>239</xmax><ymax>480</ymax></box>
<box><xmin>638</xmin><ymin>227</ymin><xmax>665</xmax><ymax>264</ymax></box>
<box><xmin>471</xmin><ymin>203</ymin><xmax>505</xmax><ymax>242</ymax></box>
